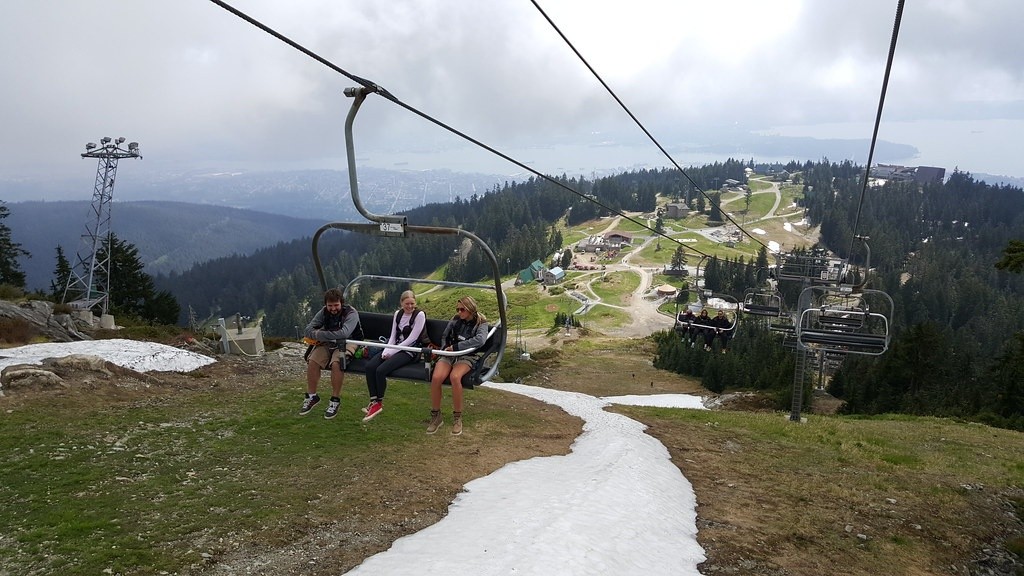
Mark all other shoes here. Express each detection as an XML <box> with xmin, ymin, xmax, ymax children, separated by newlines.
<box><xmin>691</xmin><ymin>342</ymin><xmax>695</xmax><ymax>349</ymax></box>
<box><xmin>722</xmin><ymin>348</ymin><xmax>726</xmax><ymax>355</ymax></box>
<box><xmin>681</xmin><ymin>338</ymin><xmax>685</xmax><ymax>342</ymax></box>
<box><xmin>688</xmin><ymin>338</ymin><xmax>692</xmax><ymax>342</ymax></box>
<box><xmin>706</xmin><ymin>346</ymin><xmax>711</xmax><ymax>351</ymax></box>
<box><xmin>703</xmin><ymin>344</ymin><xmax>707</xmax><ymax>350</ymax></box>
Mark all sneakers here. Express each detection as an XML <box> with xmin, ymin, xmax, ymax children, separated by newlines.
<box><xmin>324</xmin><ymin>396</ymin><xmax>341</xmax><ymax>420</ymax></box>
<box><xmin>362</xmin><ymin>401</ymin><xmax>383</xmax><ymax>423</ymax></box>
<box><xmin>299</xmin><ymin>392</ymin><xmax>322</xmax><ymax>416</ymax></box>
<box><xmin>360</xmin><ymin>401</ymin><xmax>375</xmax><ymax>412</ymax></box>
<box><xmin>450</xmin><ymin>411</ymin><xmax>462</xmax><ymax>435</ymax></box>
<box><xmin>426</xmin><ymin>410</ymin><xmax>444</xmax><ymax>434</ymax></box>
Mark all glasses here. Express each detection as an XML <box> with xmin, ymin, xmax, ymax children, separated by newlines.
<box><xmin>456</xmin><ymin>308</ymin><xmax>467</xmax><ymax>313</ymax></box>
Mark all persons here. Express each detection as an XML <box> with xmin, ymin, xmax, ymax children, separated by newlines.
<box><xmin>680</xmin><ymin>308</ymin><xmax>731</xmax><ymax>354</ymax></box>
<box><xmin>299</xmin><ymin>289</ymin><xmax>364</xmax><ymax>419</ymax></box>
<box><xmin>361</xmin><ymin>290</ymin><xmax>430</xmax><ymax>422</ymax></box>
<box><xmin>425</xmin><ymin>296</ymin><xmax>488</xmax><ymax>435</ymax></box>
